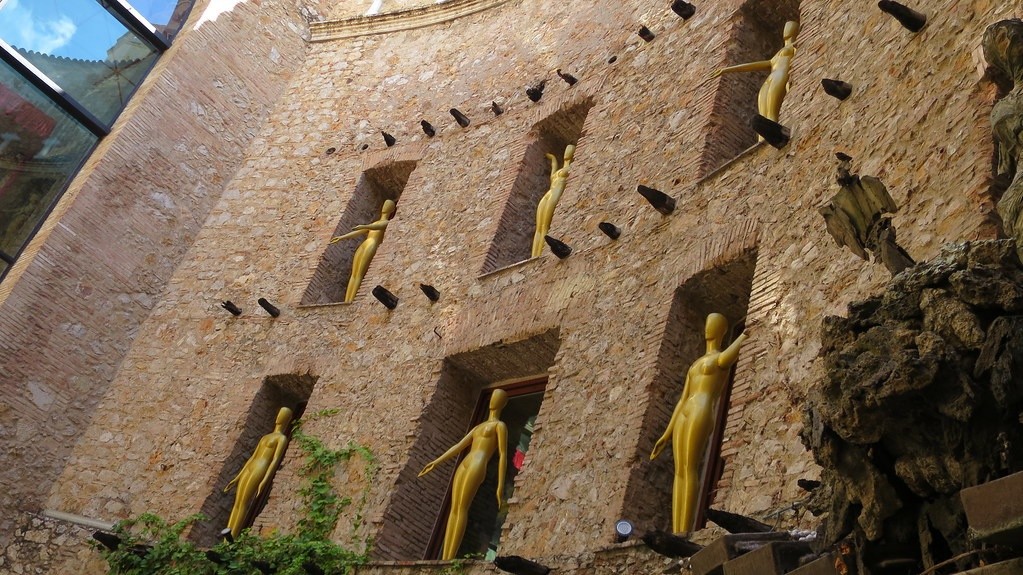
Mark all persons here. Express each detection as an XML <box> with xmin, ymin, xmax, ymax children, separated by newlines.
<box><xmin>328</xmin><ymin>199</ymin><xmax>395</xmax><ymax>303</ymax></box>
<box><xmin>712</xmin><ymin>21</ymin><xmax>799</xmax><ymax>142</ymax></box>
<box><xmin>418</xmin><ymin>389</ymin><xmax>508</xmax><ymax>561</ymax></box>
<box><xmin>531</xmin><ymin>145</ymin><xmax>575</xmax><ymax>258</ymax></box>
<box><xmin>222</xmin><ymin>406</ymin><xmax>292</xmax><ymax>544</ymax></box>
<box><xmin>651</xmin><ymin>313</ymin><xmax>747</xmax><ymax>536</ymax></box>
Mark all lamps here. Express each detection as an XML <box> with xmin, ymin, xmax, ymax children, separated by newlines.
<box><xmin>221</xmin><ymin>528</ymin><xmax>236</xmax><ymax>543</ymax></box>
<box><xmin>616</xmin><ymin>520</ymin><xmax>632</xmax><ymax>542</ymax></box>
